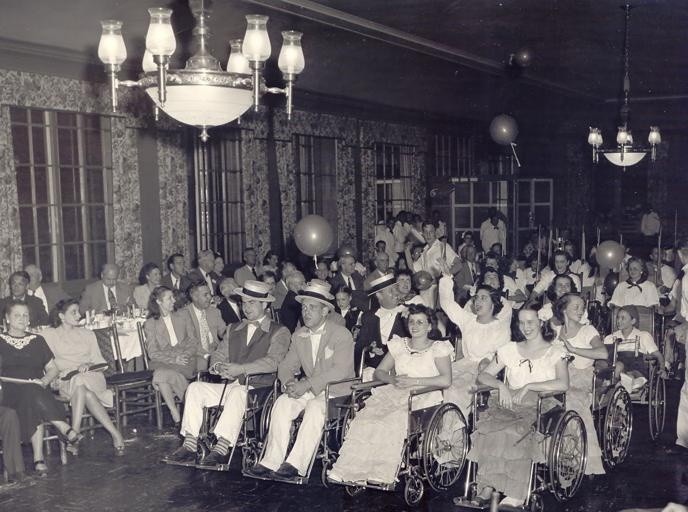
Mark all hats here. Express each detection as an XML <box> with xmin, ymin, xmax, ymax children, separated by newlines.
<box><xmin>233</xmin><ymin>280</ymin><xmax>277</xmax><ymax>302</ymax></box>
<box><xmin>306</xmin><ymin>279</ymin><xmax>335</xmax><ymax>301</ymax></box>
<box><xmin>367</xmin><ymin>273</ymin><xmax>400</xmax><ymax>297</ymax></box>
<box><xmin>295</xmin><ymin>286</ymin><xmax>335</xmax><ymax>310</ymax></box>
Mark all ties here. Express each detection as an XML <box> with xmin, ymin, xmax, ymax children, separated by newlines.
<box><xmin>108</xmin><ymin>287</ymin><xmax>119</xmax><ymax>310</ymax></box>
<box><xmin>174</xmin><ymin>280</ymin><xmax>178</xmax><ymax>288</ymax></box>
<box><xmin>252</xmin><ymin>267</ymin><xmax>258</xmax><ymax>278</ymax></box>
<box><xmin>201</xmin><ymin>312</ymin><xmax>211</xmax><ymax>352</ymax></box>
<box><xmin>32</xmin><ymin>291</ymin><xmax>36</xmax><ymax>296</ymax></box>
<box><xmin>471</xmin><ymin>264</ymin><xmax>478</xmax><ymax>283</ymax></box>
<box><xmin>347</xmin><ymin>277</ymin><xmax>353</xmax><ymax>290</ymax></box>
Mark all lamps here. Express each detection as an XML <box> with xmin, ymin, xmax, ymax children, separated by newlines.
<box><xmin>98</xmin><ymin>0</ymin><xmax>305</xmax><ymax>143</ymax></box>
<box><xmin>588</xmin><ymin>5</ymin><xmax>662</xmax><ymax>171</ymax></box>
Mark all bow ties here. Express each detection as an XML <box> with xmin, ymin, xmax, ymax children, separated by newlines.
<box><xmin>375</xmin><ymin>305</ymin><xmax>404</xmax><ymax>318</ymax></box>
<box><xmin>243</xmin><ymin>318</ymin><xmax>260</xmax><ymax>328</ymax></box>
<box><xmin>494</xmin><ymin>227</ymin><xmax>499</xmax><ymax>229</ymax></box>
<box><xmin>310</xmin><ymin>329</ymin><xmax>322</xmax><ymax>335</ymax></box>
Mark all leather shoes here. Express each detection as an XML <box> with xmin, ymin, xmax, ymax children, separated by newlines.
<box><xmin>269</xmin><ymin>462</ymin><xmax>298</xmax><ymax>480</ymax></box>
<box><xmin>164</xmin><ymin>446</ymin><xmax>197</xmax><ymax>464</ymax></box>
<box><xmin>8</xmin><ymin>472</ymin><xmax>39</xmax><ymax>487</ymax></box>
<box><xmin>199</xmin><ymin>449</ymin><xmax>230</xmax><ymax>466</ymax></box>
<box><xmin>243</xmin><ymin>464</ymin><xmax>273</xmax><ymax>477</ymax></box>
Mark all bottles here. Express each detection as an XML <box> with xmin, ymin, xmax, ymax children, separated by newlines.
<box><xmin>85</xmin><ymin>309</ymin><xmax>99</xmax><ymax>331</ymax></box>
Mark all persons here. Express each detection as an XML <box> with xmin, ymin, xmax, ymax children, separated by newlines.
<box><xmin>550</xmin><ymin>292</ymin><xmax>608</xmax><ymax>475</ymax></box>
<box><xmin>376</xmin><ymin>211</ymin><xmax>448</xmax><ymax>268</ymax></box>
<box><xmin>458</xmin><ymin>207</ymin><xmax>506</xmax><ymax>258</ymax></box>
<box><xmin>465</xmin><ymin>301</ymin><xmax>570</xmax><ymax>508</ymax></box>
<box><xmin>0</xmin><ymin>300</ymin><xmax>78</xmax><ymax>476</ymax></box>
<box><xmin>162</xmin><ymin>279</ymin><xmax>291</xmax><ymax>467</ymax></box>
<box><xmin>241</xmin><ymin>286</ymin><xmax>354</xmax><ymax>481</ymax></box>
<box><xmin>325</xmin><ymin>305</ymin><xmax>453</xmax><ymax>488</ymax></box>
<box><xmin>0</xmin><ymin>406</ymin><xmax>38</xmax><ymax>488</ymax></box>
<box><xmin>533</xmin><ymin>225</ymin><xmax>570</xmax><ymax>254</ymax></box>
<box><xmin>641</xmin><ymin>207</ymin><xmax>659</xmax><ymax>254</ymax></box>
<box><xmin>0</xmin><ymin>240</ymin><xmax>688</xmax><ymax>393</ymax></box>
<box><xmin>672</xmin><ymin>258</ymin><xmax>687</xmax><ymax>451</ymax></box>
<box><xmin>40</xmin><ymin>301</ymin><xmax>126</xmax><ymax>458</ymax></box>
<box><xmin>141</xmin><ymin>285</ymin><xmax>198</xmax><ymax>436</ymax></box>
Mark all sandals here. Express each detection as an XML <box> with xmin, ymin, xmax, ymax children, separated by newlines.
<box><xmin>113</xmin><ymin>441</ymin><xmax>126</xmax><ymax>456</ymax></box>
<box><xmin>63</xmin><ymin>428</ymin><xmax>86</xmax><ymax>446</ymax></box>
<box><xmin>469</xmin><ymin>496</ymin><xmax>491</xmax><ymax>507</ymax></box>
<box><xmin>66</xmin><ymin>445</ymin><xmax>79</xmax><ymax>456</ymax></box>
<box><xmin>33</xmin><ymin>457</ymin><xmax>49</xmax><ymax>478</ymax></box>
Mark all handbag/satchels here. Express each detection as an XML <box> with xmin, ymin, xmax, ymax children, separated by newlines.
<box><xmin>149</xmin><ymin>344</ymin><xmax>197</xmax><ymax>379</ymax></box>
<box><xmin>49</xmin><ymin>363</ymin><xmax>110</xmax><ymax>391</ymax></box>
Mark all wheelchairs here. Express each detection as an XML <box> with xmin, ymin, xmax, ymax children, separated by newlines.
<box><xmin>164</xmin><ymin>344</ymin><xmax>281</xmax><ymax>474</ymax></box>
<box><xmin>591</xmin><ymin>338</ymin><xmax>633</xmax><ymax>466</ymax></box>
<box><xmin>452</xmin><ymin>384</ymin><xmax>586</xmax><ymax>511</ymax></box>
<box><xmin>606</xmin><ymin>306</ymin><xmax>667</xmax><ymax>439</ymax></box>
<box><xmin>246</xmin><ymin>345</ymin><xmax>368</xmax><ymax>488</ymax></box>
<box><xmin>343</xmin><ymin>375</ymin><xmax>469</xmax><ymax>507</ymax></box>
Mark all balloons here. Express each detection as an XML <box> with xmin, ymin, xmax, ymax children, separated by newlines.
<box><xmin>487</xmin><ymin>113</ymin><xmax>519</xmax><ymax>145</ymax></box>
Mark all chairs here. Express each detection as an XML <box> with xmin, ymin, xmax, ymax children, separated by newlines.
<box><xmin>20</xmin><ymin>322</ymin><xmax>185</xmax><ymax>465</ymax></box>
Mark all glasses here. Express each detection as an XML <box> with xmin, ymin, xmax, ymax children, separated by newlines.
<box><xmin>408</xmin><ymin>318</ymin><xmax>429</xmax><ymax>326</ymax></box>
